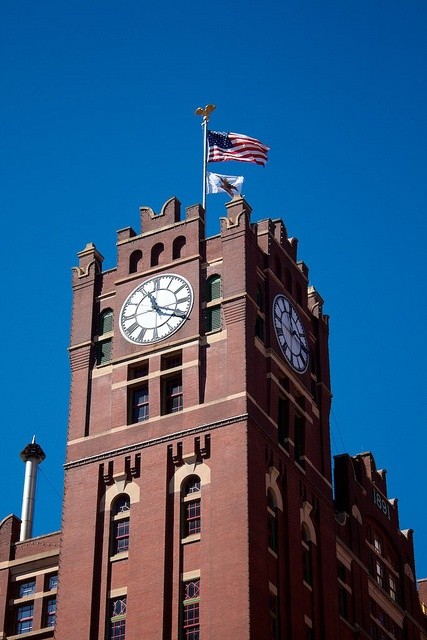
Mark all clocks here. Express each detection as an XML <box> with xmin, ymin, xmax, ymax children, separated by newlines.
<box><xmin>271</xmin><ymin>294</ymin><xmax>310</xmax><ymax>375</ymax></box>
<box><xmin>118</xmin><ymin>272</ymin><xmax>194</xmax><ymax>346</ymax></box>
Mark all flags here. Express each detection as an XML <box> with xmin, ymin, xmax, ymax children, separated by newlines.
<box><xmin>206</xmin><ymin>172</ymin><xmax>245</xmax><ymax>200</ymax></box>
<box><xmin>206</xmin><ymin>130</ymin><xmax>270</xmax><ymax>168</ymax></box>
